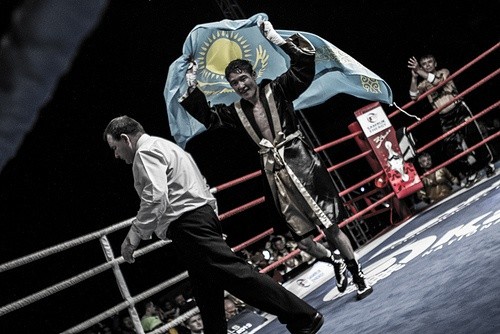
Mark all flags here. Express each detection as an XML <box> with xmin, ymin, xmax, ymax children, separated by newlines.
<box><xmin>164</xmin><ymin>12</ymin><xmax>393</xmax><ymax>149</ymax></box>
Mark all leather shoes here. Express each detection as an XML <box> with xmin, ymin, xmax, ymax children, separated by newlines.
<box><xmin>291</xmin><ymin>311</ymin><xmax>324</xmax><ymax>334</ymax></box>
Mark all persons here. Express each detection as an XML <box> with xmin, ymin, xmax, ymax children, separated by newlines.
<box><xmin>178</xmin><ymin>22</ymin><xmax>373</xmax><ymax>301</ymax></box>
<box><xmin>103</xmin><ymin>116</ymin><xmax>324</xmax><ymax>334</ymax></box>
<box><xmin>416</xmin><ymin>153</ymin><xmax>458</xmax><ymax>205</ymax></box>
<box><xmin>407</xmin><ymin>55</ymin><xmax>496</xmax><ymax>188</ymax></box>
<box><xmin>97</xmin><ymin>229</ymin><xmax>317</xmax><ymax>334</ymax></box>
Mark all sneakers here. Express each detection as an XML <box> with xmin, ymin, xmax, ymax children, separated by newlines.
<box><xmin>466</xmin><ymin>172</ymin><xmax>481</xmax><ymax>188</ymax></box>
<box><xmin>486</xmin><ymin>163</ymin><xmax>495</xmax><ymax>178</ymax></box>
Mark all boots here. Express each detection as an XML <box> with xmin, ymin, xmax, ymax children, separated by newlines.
<box><xmin>344</xmin><ymin>256</ymin><xmax>373</xmax><ymax>300</ymax></box>
<box><xmin>319</xmin><ymin>248</ymin><xmax>348</xmax><ymax>292</ymax></box>
